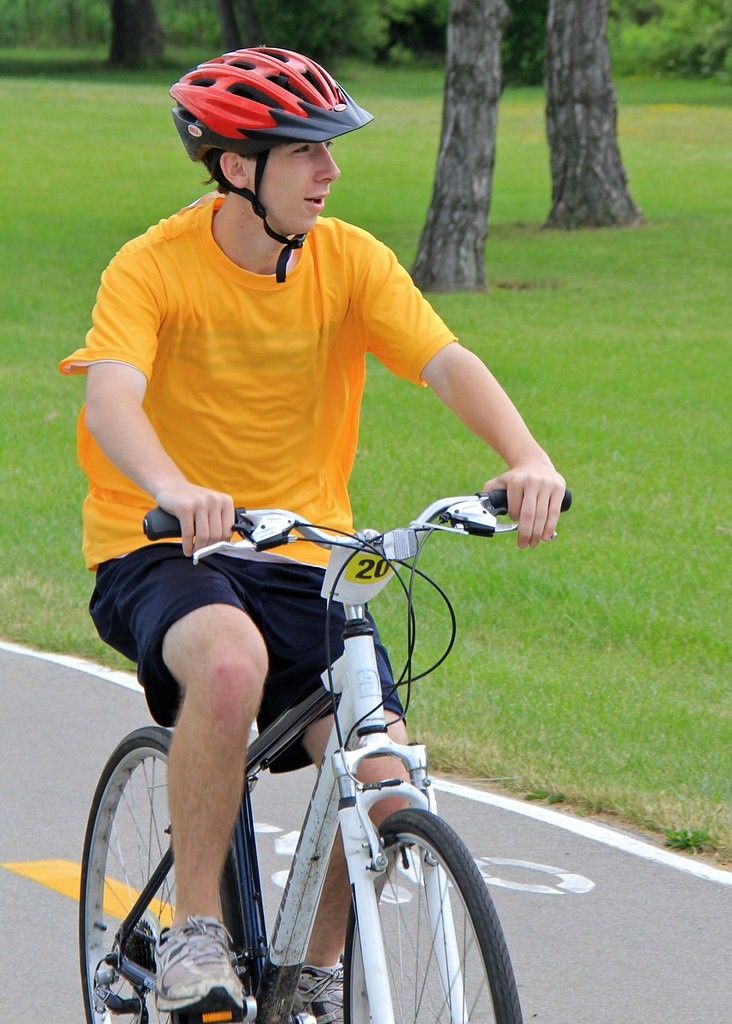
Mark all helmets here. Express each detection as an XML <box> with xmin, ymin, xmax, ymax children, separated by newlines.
<box><xmin>170</xmin><ymin>48</ymin><xmax>374</xmax><ymax>162</ymax></box>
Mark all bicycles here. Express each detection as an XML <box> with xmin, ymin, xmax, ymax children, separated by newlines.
<box><xmin>74</xmin><ymin>488</ymin><xmax>580</xmax><ymax>1024</ymax></box>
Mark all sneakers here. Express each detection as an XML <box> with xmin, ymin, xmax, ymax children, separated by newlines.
<box><xmin>295</xmin><ymin>954</ymin><xmax>346</xmax><ymax>1024</ymax></box>
<box><xmin>154</xmin><ymin>914</ymin><xmax>244</xmax><ymax>1015</ymax></box>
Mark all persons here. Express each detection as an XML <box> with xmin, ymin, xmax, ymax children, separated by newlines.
<box><xmin>57</xmin><ymin>41</ymin><xmax>566</xmax><ymax>1024</ymax></box>
